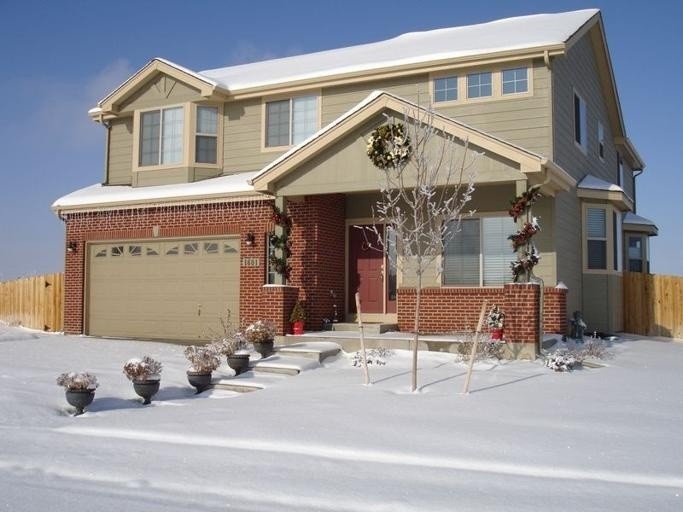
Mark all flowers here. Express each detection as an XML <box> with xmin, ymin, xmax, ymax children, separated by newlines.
<box><xmin>263</xmin><ymin>206</ymin><xmax>293</xmax><ymax>280</ymax></box>
<box><xmin>364</xmin><ymin>122</ymin><xmax>414</xmax><ymax>169</ymax></box>
<box><xmin>505</xmin><ymin>186</ymin><xmax>545</xmax><ymax>284</ymax></box>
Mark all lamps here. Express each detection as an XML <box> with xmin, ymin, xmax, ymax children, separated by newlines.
<box><xmin>65</xmin><ymin>240</ymin><xmax>76</xmax><ymax>254</ymax></box>
<box><xmin>243</xmin><ymin>232</ymin><xmax>256</xmax><ymax>246</ymax></box>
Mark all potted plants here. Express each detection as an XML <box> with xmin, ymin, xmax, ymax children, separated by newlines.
<box><xmin>56</xmin><ymin>369</ymin><xmax>99</xmax><ymax>417</ymax></box>
<box><xmin>182</xmin><ymin>343</ymin><xmax>218</xmax><ymax>395</ymax></box>
<box><xmin>486</xmin><ymin>302</ymin><xmax>505</xmax><ymax>340</ymax></box>
<box><xmin>120</xmin><ymin>353</ymin><xmax>163</xmax><ymax>406</ymax></box>
<box><xmin>215</xmin><ymin>331</ymin><xmax>248</xmax><ymax>378</ymax></box>
<box><xmin>287</xmin><ymin>298</ymin><xmax>306</xmax><ymax>335</ymax></box>
<box><xmin>244</xmin><ymin>318</ymin><xmax>276</xmax><ymax>360</ymax></box>
